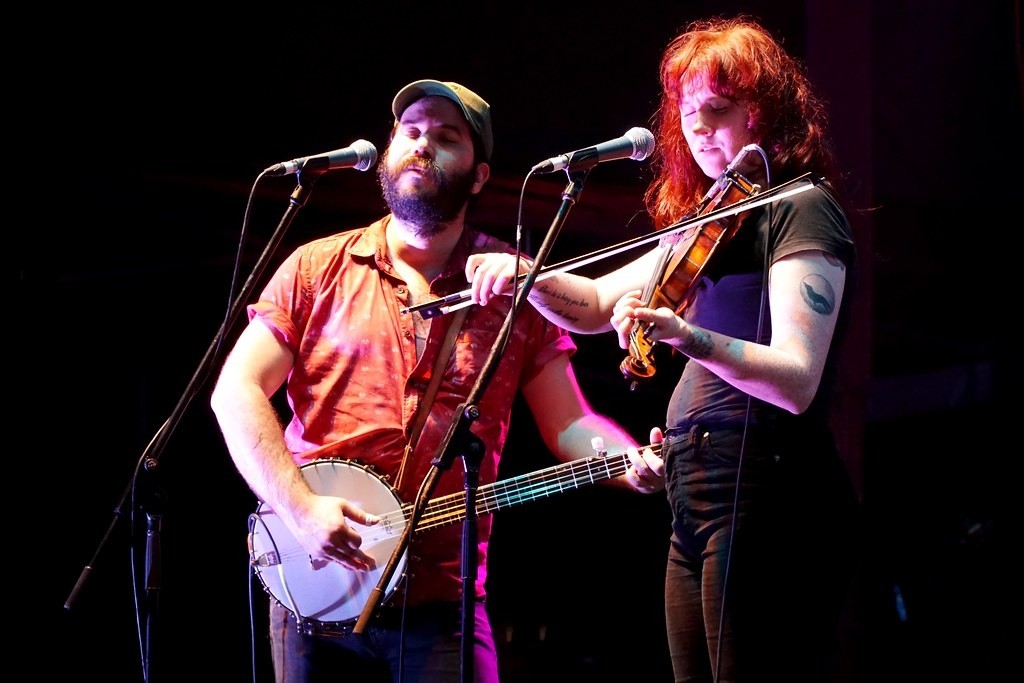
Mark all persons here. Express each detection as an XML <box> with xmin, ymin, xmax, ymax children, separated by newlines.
<box><xmin>466</xmin><ymin>16</ymin><xmax>859</xmax><ymax>683</ymax></box>
<box><xmin>210</xmin><ymin>80</ymin><xmax>666</xmax><ymax>683</ymax></box>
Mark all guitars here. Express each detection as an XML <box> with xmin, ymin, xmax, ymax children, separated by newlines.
<box><xmin>244</xmin><ymin>439</ymin><xmax>666</xmax><ymax>630</ymax></box>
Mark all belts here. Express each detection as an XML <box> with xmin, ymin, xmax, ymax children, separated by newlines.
<box><xmin>662</xmin><ymin>426</ymin><xmax>730</xmax><ymax>447</ymax></box>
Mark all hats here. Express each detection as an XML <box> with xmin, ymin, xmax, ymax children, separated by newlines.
<box><xmin>392</xmin><ymin>80</ymin><xmax>491</xmax><ymax>160</ymax></box>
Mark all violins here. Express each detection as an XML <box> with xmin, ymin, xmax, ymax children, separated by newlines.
<box><xmin>620</xmin><ymin>168</ymin><xmax>761</xmax><ymax>395</ymax></box>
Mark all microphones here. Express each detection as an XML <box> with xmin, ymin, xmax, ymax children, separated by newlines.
<box><xmin>265</xmin><ymin>140</ymin><xmax>378</xmax><ymax>177</ymax></box>
<box><xmin>533</xmin><ymin>128</ymin><xmax>656</xmax><ymax>175</ymax></box>
